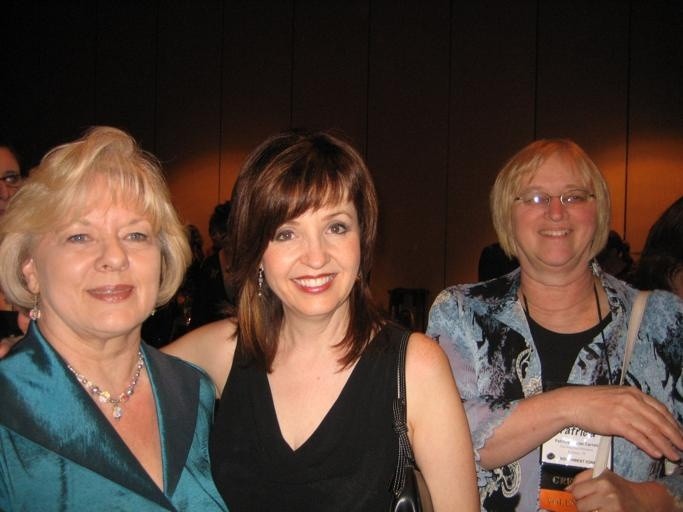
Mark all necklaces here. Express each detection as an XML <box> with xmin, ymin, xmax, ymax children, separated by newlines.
<box><xmin>64</xmin><ymin>350</ymin><xmax>146</xmax><ymax>420</ymax></box>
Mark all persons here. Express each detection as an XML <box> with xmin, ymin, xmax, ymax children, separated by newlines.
<box><xmin>422</xmin><ymin>139</ymin><xmax>683</xmax><ymax>512</ymax></box>
<box><xmin>0</xmin><ymin>124</ymin><xmax>228</xmax><ymax>512</ymax></box>
<box><xmin>139</xmin><ymin>199</ymin><xmax>234</xmax><ymax>345</ymax></box>
<box><xmin>633</xmin><ymin>195</ymin><xmax>683</xmax><ymax>297</ymax></box>
<box><xmin>0</xmin><ymin>145</ymin><xmax>27</xmax><ymax>335</ymax></box>
<box><xmin>0</xmin><ymin>130</ymin><xmax>480</xmax><ymax>511</ymax></box>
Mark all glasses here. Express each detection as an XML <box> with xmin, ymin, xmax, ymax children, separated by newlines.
<box><xmin>509</xmin><ymin>188</ymin><xmax>598</xmax><ymax>208</ymax></box>
